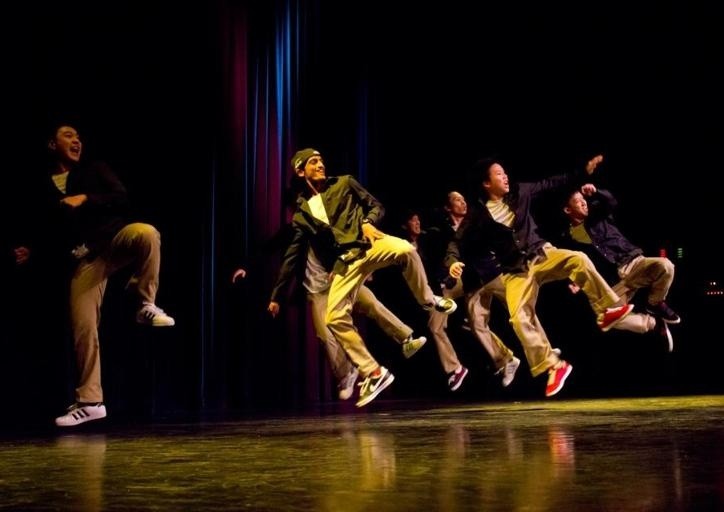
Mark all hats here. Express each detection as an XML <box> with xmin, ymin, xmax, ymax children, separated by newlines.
<box><xmin>291</xmin><ymin>148</ymin><xmax>320</xmax><ymax>169</ymax></box>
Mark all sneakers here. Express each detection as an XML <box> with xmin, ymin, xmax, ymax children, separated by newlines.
<box><xmin>337</xmin><ymin>367</ymin><xmax>359</xmax><ymax>400</ymax></box>
<box><xmin>355</xmin><ymin>366</ymin><xmax>395</xmax><ymax>408</ymax></box>
<box><xmin>423</xmin><ymin>295</ymin><xmax>457</xmax><ymax>315</ymax></box>
<box><xmin>597</xmin><ymin>304</ymin><xmax>634</xmax><ymax>332</ymax></box>
<box><xmin>545</xmin><ymin>360</ymin><xmax>573</xmax><ymax>396</ymax></box>
<box><xmin>646</xmin><ymin>301</ymin><xmax>680</xmax><ymax>323</ymax></box>
<box><xmin>654</xmin><ymin>316</ymin><xmax>673</xmax><ymax>352</ymax></box>
<box><xmin>448</xmin><ymin>365</ymin><xmax>469</xmax><ymax>391</ymax></box>
<box><xmin>403</xmin><ymin>336</ymin><xmax>427</xmax><ymax>358</ymax></box>
<box><xmin>495</xmin><ymin>356</ymin><xmax>521</xmax><ymax>387</ymax></box>
<box><xmin>136</xmin><ymin>303</ymin><xmax>175</xmax><ymax>327</ymax></box>
<box><xmin>55</xmin><ymin>403</ymin><xmax>107</xmax><ymax>427</ymax></box>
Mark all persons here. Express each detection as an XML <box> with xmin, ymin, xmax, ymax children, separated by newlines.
<box><xmin>266</xmin><ymin>147</ymin><xmax>458</xmax><ymax>409</ymax></box>
<box><xmin>13</xmin><ymin>123</ymin><xmax>176</xmax><ymax>428</ymax></box>
<box><xmin>442</xmin><ymin>156</ymin><xmax>636</xmax><ymax>399</ymax></box>
<box><xmin>554</xmin><ymin>183</ymin><xmax>683</xmax><ymax>352</ymax></box>
<box><xmin>440</xmin><ymin>188</ymin><xmax>522</xmax><ymax>396</ymax></box>
<box><xmin>229</xmin><ymin>175</ymin><xmax>427</xmax><ymax>403</ymax></box>
<box><xmin>365</xmin><ymin>211</ymin><xmax>469</xmax><ymax>391</ymax></box>
